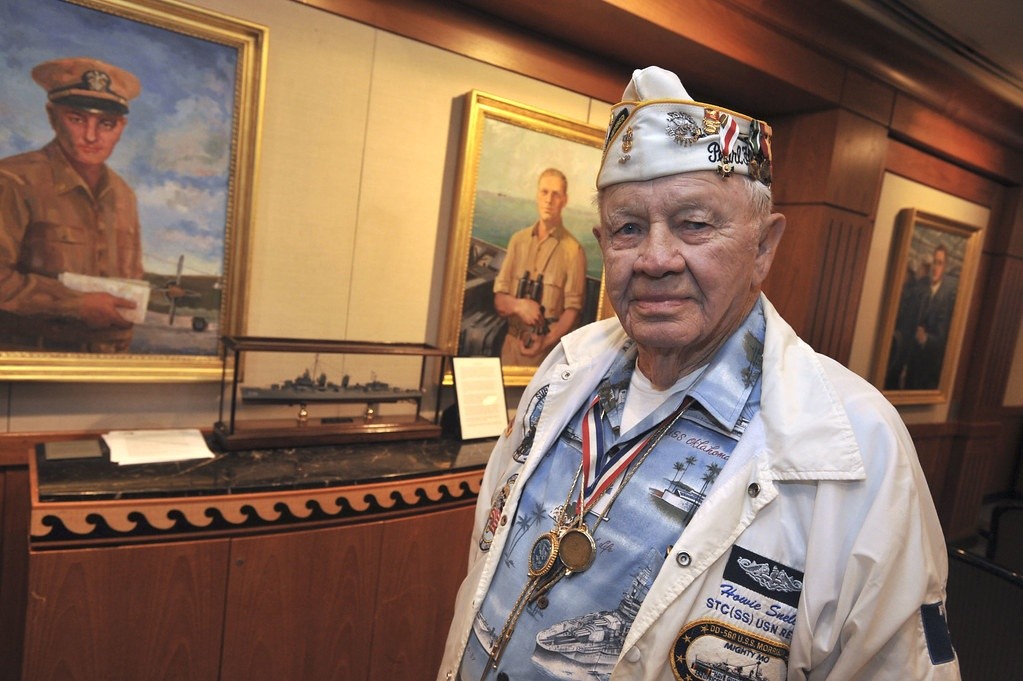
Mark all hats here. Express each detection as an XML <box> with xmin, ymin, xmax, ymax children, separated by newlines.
<box><xmin>595</xmin><ymin>66</ymin><xmax>774</xmax><ymax>189</ymax></box>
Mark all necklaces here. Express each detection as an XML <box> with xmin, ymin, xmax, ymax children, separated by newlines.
<box><xmin>479</xmin><ymin>367</ymin><xmax>698</xmax><ymax>680</ymax></box>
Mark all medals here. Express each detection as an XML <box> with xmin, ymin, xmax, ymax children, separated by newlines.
<box><xmin>557</xmin><ymin>466</ymin><xmax>621</xmax><ymax>577</ymax></box>
<box><xmin>524</xmin><ymin>379</ymin><xmax>675</xmax><ymax>577</ymax></box>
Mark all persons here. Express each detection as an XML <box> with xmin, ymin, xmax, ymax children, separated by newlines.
<box><xmin>436</xmin><ymin>62</ymin><xmax>962</xmax><ymax>681</ymax></box>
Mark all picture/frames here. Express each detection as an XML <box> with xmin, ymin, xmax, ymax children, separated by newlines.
<box><xmin>871</xmin><ymin>208</ymin><xmax>984</xmax><ymax>405</ymax></box>
<box><xmin>432</xmin><ymin>90</ymin><xmax>610</xmax><ymax>384</ymax></box>
<box><xmin>0</xmin><ymin>2</ymin><xmax>268</xmax><ymax>383</ymax></box>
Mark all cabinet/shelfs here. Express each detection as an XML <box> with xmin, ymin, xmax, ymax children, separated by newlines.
<box><xmin>23</xmin><ymin>503</ymin><xmax>477</xmax><ymax>681</ymax></box>
